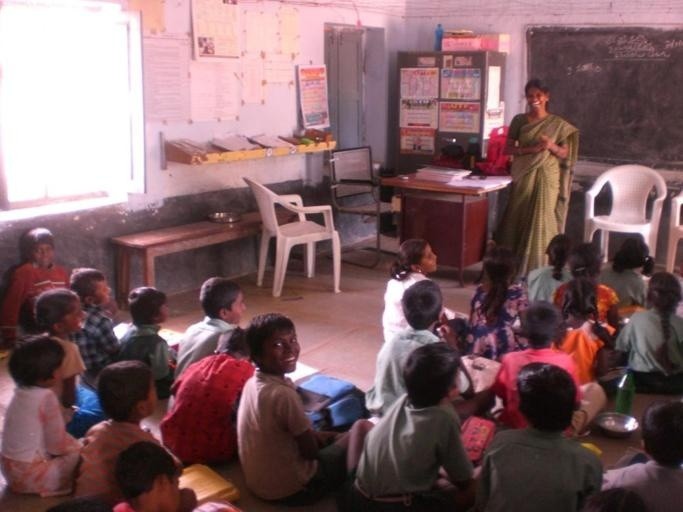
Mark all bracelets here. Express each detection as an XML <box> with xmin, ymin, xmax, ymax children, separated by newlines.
<box><xmin>551</xmin><ymin>145</ymin><xmax>559</xmax><ymax>155</ymax></box>
<box><xmin>517</xmin><ymin>148</ymin><xmax>523</xmax><ymax>156</ymax></box>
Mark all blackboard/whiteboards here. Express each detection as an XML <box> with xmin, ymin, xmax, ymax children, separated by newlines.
<box><xmin>526</xmin><ymin>26</ymin><xmax>681</xmax><ymax>170</ymax></box>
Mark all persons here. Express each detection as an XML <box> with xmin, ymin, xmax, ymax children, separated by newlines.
<box><xmin>36</xmin><ymin>289</ymin><xmax>110</xmax><ymax>438</ymax></box>
<box><xmin>70</xmin><ymin>265</ymin><xmax>122</xmax><ymax>377</ymax></box>
<box><xmin>159</xmin><ymin>327</ymin><xmax>255</xmax><ymax>467</ymax></box>
<box><xmin>119</xmin><ymin>284</ymin><xmax>171</xmax><ymax>399</ymax></box>
<box><xmin>0</xmin><ymin>226</ymin><xmax>69</xmax><ymax>348</ymax></box>
<box><xmin>174</xmin><ymin>276</ymin><xmax>247</xmax><ymax>379</ymax></box>
<box><xmin>1</xmin><ymin>333</ymin><xmax>85</xmax><ymax>499</ymax></box>
<box><xmin>493</xmin><ymin>78</ymin><xmax>580</xmax><ymax>278</ymax></box>
<box><xmin>112</xmin><ymin>440</ymin><xmax>197</xmax><ymax>512</ymax></box>
<box><xmin>236</xmin><ymin>312</ymin><xmax>350</xmax><ymax>505</ymax></box>
<box><xmin>345</xmin><ymin>232</ymin><xmax>682</xmax><ymax>511</ymax></box>
<box><xmin>73</xmin><ymin>359</ymin><xmax>184</xmax><ymax>512</ymax></box>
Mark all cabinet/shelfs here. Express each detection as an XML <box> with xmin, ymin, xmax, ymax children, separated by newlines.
<box><xmin>398</xmin><ymin>50</ymin><xmax>507</xmax><ymax>159</ymax></box>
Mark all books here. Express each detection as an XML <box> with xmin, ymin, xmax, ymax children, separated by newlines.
<box><xmin>211</xmin><ymin>130</ymin><xmax>296</xmax><ymax>152</ymax></box>
<box><xmin>414</xmin><ymin>164</ymin><xmax>472</xmax><ymax>183</ymax></box>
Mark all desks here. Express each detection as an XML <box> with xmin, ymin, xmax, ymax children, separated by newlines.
<box><xmin>381</xmin><ymin>166</ymin><xmax>513</xmax><ymax>288</ymax></box>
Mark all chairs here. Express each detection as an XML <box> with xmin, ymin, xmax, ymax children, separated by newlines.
<box><xmin>584</xmin><ymin>165</ymin><xmax>667</xmax><ymax>263</ymax></box>
<box><xmin>327</xmin><ymin>145</ymin><xmax>401</xmax><ymax>268</ymax></box>
<box><xmin>666</xmin><ymin>188</ymin><xmax>682</xmax><ymax>273</ymax></box>
<box><xmin>243</xmin><ymin>175</ymin><xmax>342</xmax><ymax>297</ymax></box>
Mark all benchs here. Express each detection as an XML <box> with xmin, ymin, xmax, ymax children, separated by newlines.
<box><xmin>111</xmin><ymin>203</ymin><xmax>315</xmax><ymax>308</ymax></box>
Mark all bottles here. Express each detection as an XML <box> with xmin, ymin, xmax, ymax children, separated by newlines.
<box><xmin>614</xmin><ymin>367</ymin><xmax>636</xmax><ymax>414</ymax></box>
<box><xmin>434</xmin><ymin>24</ymin><xmax>443</xmax><ymax>51</ymax></box>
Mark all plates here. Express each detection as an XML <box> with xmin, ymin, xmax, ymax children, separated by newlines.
<box><xmin>209</xmin><ymin>211</ymin><xmax>240</xmax><ymax>223</ymax></box>
<box><xmin>593</xmin><ymin>411</ymin><xmax>638</xmax><ymax>435</ymax></box>
